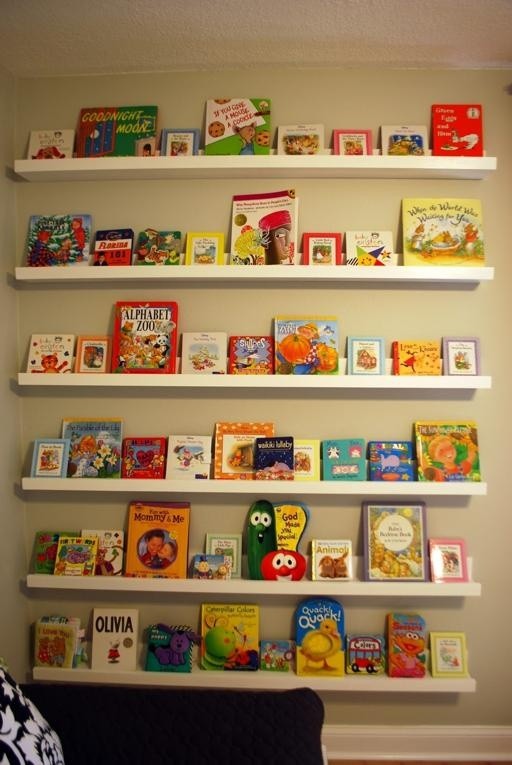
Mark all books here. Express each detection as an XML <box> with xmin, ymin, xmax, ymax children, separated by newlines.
<box><xmin>23</xmin><ymin>189</ymin><xmax>487</xmax><ymax>266</ymax></box>
<box><xmin>24</xmin><ymin>299</ymin><xmax>479</xmax><ymax>374</ymax></box>
<box><xmin>32</xmin><ymin>416</ymin><xmax>482</xmax><ymax>483</ymax></box>
<box><xmin>23</xmin><ymin>94</ymin><xmax>486</xmax><ymax>160</ymax></box>
<box><xmin>33</xmin><ymin>595</ymin><xmax>467</xmax><ymax>679</ymax></box>
<box><xmin>32</xmin><ymin>499</ymin><xmax>475</xmax><ymax>582</ymax></box>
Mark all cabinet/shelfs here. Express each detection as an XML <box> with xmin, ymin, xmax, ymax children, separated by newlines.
<box><xmin>14</xmin><ymin>155</ymin><xmax>499</xmax><ymax>693</ymax></box>
